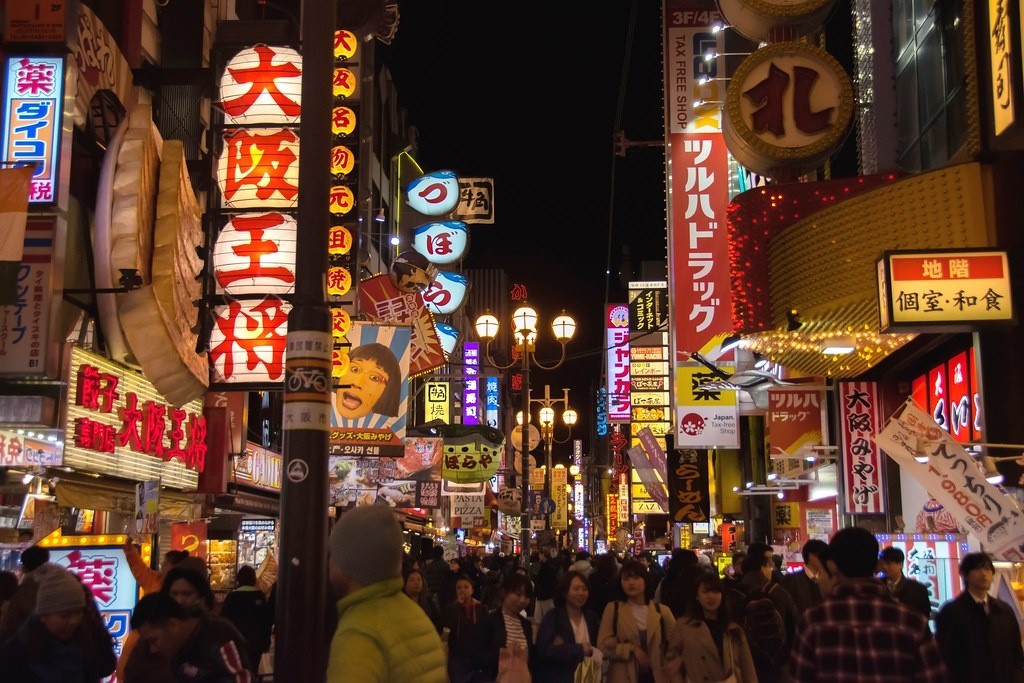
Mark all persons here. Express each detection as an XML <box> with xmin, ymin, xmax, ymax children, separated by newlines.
<box><xmin>435</xmin><ymin>575</ymin><xmax>490</xmax><ymax>650</ymax></box>
<box><xmin>0</xmin><ymin>502</ymin><xmax>1024</xmax><ymax>683</ymax></box>
<box><xmin>874</xmin><ymin>548</ymin><xmax>932</xmax><ymax>619</ymax></box>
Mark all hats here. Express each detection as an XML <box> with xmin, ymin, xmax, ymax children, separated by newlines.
<box><xmin>37</xmin><ymin>567</ymin><xmax>87</xmax><ymax>614</ymax></box>
<box><xmin>329</xmin><ymin>505</ymin><xmax>404</xmax><ymax>585</ymax></box>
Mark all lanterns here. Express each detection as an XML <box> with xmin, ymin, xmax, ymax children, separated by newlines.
<box><xmin>326</xmin><ymin>267</ymin><xmax>352</xmax><ymax>296</ymax></box>
<box><xmin>328</xmin><ymin>225</ymin><xmax>352</xmax><ymax>258</ymax></box>
<box><xmin>331</xmin><ymin>106</ymin><xmax>356</xmax><ymax>139</ymax></box>
<box><xmin>332</xmin><ymin>350</ymin><xmax>349</xmax><ymax>378</ymax></box>
<box><xmin>330</xmin><ymin>145</ymin><xmax>354</xmax><ymax>181</ymax></box>
<box><xmin>329</xmin><ymin>186</ymin><xmax>355</xmax><ymax>217</ymax></box>
<box><xmin>217</xmin><ymin>44</ymin><xmax>302</xmax><ymax>121</ymax></box>
<box><xmin>215</xmin><ymin>127</ymin><xmax>300</xmax><ymax>205</ymax></box>
<box><xmin>333</xmin><ymin>29</ymin><xmax>357</xmax><ymax>61</ymax></box>
<box><xmin>210</xmin><ymin>300</ymin><xmax>292</xmax><ymax>383</ymax></box>
<box><xmin>213</xmin><ymin>215</ymin><xmax>297</xmax><ymax>294</ymax></box>
<box><xmin>333</xmin><ymin>67</ymin><xmax>356</xmax><ymax>98</ymax></box>
<box><xmin>328</xmin><ymin>307</ymin><xmax>350</xmax><ymax>341</ymax></box>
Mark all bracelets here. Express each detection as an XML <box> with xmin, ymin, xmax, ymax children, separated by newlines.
<box><xmin>631</xmin><ymin>645</ymin><xmax>640</xmax><ymax>653</ymax></box>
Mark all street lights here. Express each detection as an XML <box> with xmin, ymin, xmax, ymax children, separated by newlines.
<box><xmin>475</xmin><ymin>304</ymin><xmax>578</xmax><ymax>557</ymax></box>
<box><xmin>515</xmin><ymin>406</ymin><xmax>577</xmax><ymax>527</ymax></box>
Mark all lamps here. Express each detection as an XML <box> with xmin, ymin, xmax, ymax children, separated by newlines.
<box><xmin>361</xmin><ymin>231</ymin><xmax>399</xmax><ymax>245</ymax></box>
<box><xmin>821</xmin><ymin>335</ymin><xmax>857</xmax><ymax>354</ymax></box>
<box><xmin>731</xmin><ymin>443</ymin><xmax>838</xmax><ymax>499</ymax></box>
<box><xmin>430</xmin><ymin>427</ymin><xmax>442</xmax><ymax>437</ymax></box>
<box><xmin>361</xmin><ymin>208</ymin><xmax>385</xmax><ymax>221</ymax></box>
<box><xmin>785</xmin><ymin>310</ymin><xmax>803</xmax><ymax>332</ymax></box>
<box><xmin>914</xmin><ymin>437</ymin><xmax>1024</xmax><ymax>484</ymax></box>
